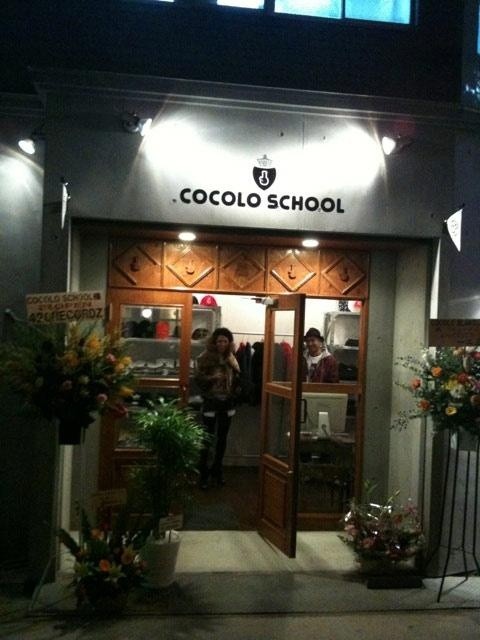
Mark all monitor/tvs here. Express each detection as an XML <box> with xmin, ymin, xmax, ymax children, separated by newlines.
<box><xmin>300</xmin><ymin>391</ymin><xmax>348</xmax><ymax>440</ymax></box>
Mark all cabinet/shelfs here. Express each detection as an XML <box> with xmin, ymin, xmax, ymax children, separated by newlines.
<box><xmin>323</xmin><ymin>312</ymin><xmax>361</xmax><ymax>421</ymax></box>
<box><xmin>120</xmin><ymin>305</ymin><xmax>221</xmax><ymax>412</ymax></box>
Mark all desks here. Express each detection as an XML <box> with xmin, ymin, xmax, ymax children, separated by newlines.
<box><xmin>287</xmin><ymin>432</ymin><xmax>355</xmax><ymax>513</ymax></box>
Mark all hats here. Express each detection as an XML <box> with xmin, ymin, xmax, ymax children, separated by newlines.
<box><xmin>302</xmin><ymin>328</ymin><xmax>325</xmax><ymax>341</ymax></box>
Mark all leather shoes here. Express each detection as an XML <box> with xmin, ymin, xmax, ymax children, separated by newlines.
<box><xmin>200</xmin><ymin>479</ymin><xmax>209</xmax><ymax>490</ymax></box>
<box><xmin>211</xmin><ymin>478</ymin><xmax>226</xmax><ymax>486</ymax></box>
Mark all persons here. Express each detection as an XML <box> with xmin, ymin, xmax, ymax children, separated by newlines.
<box><xmin>127</xmin><ymin>297</ymin><xmax>241</xmax><ymax>486</ymax></box>
<box><xmin>300</xmin><ymin>327</ymin><xmax>340</xmax><ymax>384</ymax></box>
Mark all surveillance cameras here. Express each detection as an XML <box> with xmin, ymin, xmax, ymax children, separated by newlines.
<box><xmin>252</xmin><ymin>295</ymin><xmax>274</xmax><ymax>305</ymax></box>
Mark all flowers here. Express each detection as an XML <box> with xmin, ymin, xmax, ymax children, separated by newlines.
<box><xmin>49</xmin><ymin>503</ymin><xmax>155</xmax><ymax>590</ymax></box>
<box><xmin>391</xmin><ymin>347</ymin><xmax>480</xmax><ymax>429</ymax></box>
<box><xmin>0</xmin><ymin>317</ymin><xmax>134</xmax><ymax>423</ymax></box>
<box><xmin>336</xmin><ymin>480</ymin><xmax>427</xmax><ymax>563</ymax></box>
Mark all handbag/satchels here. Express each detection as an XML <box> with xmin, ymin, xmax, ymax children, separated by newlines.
<box><xmin>231</xmin><ymin>372</ymin><xmax>260</xmax><ymax>407</ymax></box>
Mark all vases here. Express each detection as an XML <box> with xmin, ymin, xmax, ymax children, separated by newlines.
<box><xmin>368</xmin><ymin>561</ymin><xmax>408</xmax><ymax>587</ymax></box>
<box><xmin>83</xmin><ymin>589</ymin><xmax>130</xmax><ymax>619</ymax></box>
<box><xmin>58</xmin><ymin>420</ymin><xmax>82</xmax><ymax>445</ymax></box>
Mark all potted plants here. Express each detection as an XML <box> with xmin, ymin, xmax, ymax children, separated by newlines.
<box><xmin>126</xmin><ymin>399</ymin><xmax>209</xmax><ymax>589</ymax></box>
